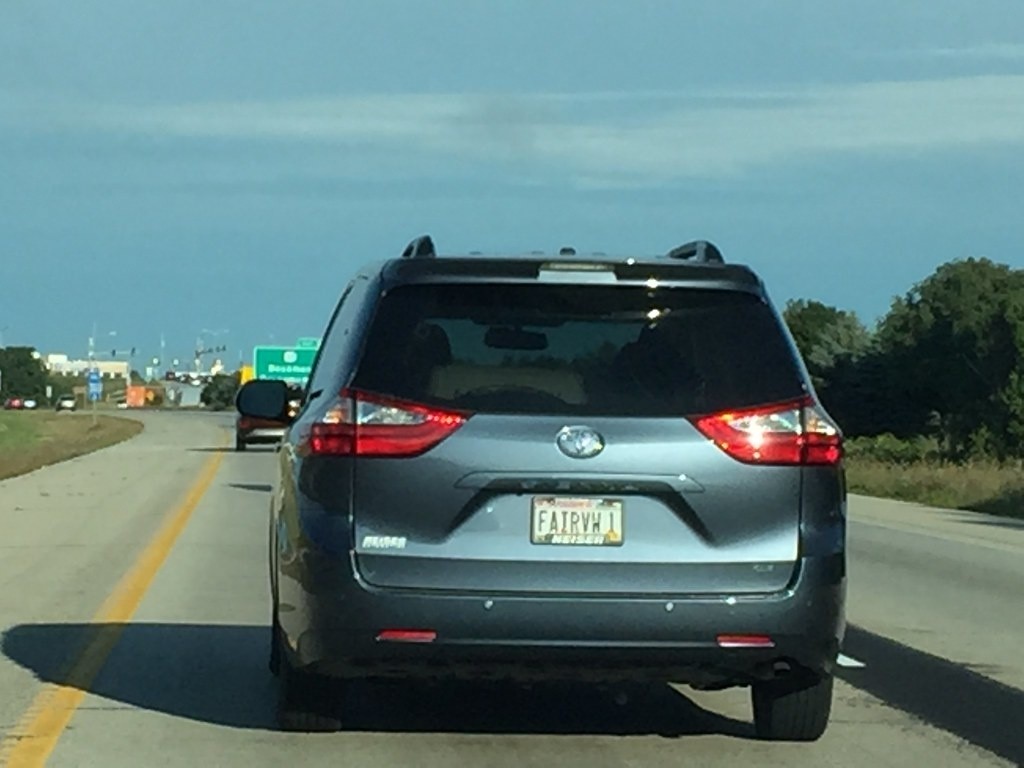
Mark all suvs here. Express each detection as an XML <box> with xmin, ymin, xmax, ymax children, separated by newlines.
<box><xmin>56</xmin><ymin>395</ymin><xmax>76</xmax><ymax>411</ymax></box>
<box><xmin>236</xmin><ymin>383</ymin><xmax>305</xmax><ymax>450</ymax></box>
<box><xmin>275</xmin><ymin>236</ymin><xmax>845</xmax><ymax>741</ymax></box>
<box><xmin>4</xmin><ymin>397</ymin><xmax>37</xmax><ymax>409</ymax></box>
